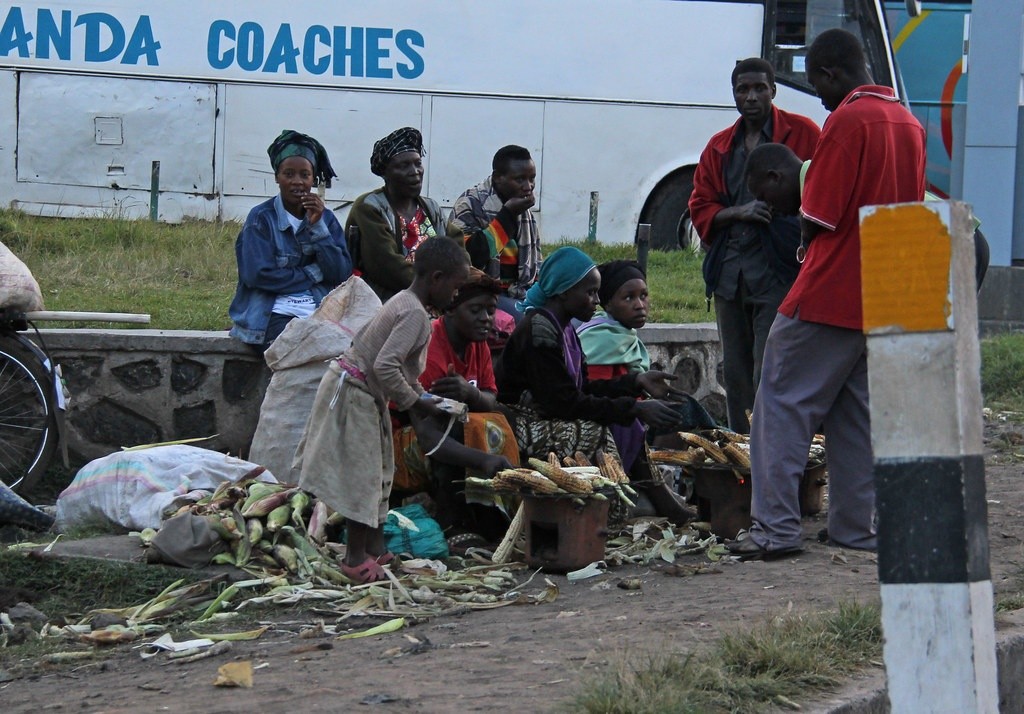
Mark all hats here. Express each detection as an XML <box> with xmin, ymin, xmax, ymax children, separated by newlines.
<box><xmin>370</xmin><ymin>126</ymin><xmax>427</xmax><ymax>176</ymax></box>
<box><xmin>266</xmin><ymin>129</ymin><xmax>337</xmax><ymax>189</ymax></box>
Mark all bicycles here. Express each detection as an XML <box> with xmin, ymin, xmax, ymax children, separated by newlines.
<box><xmin>0</xmin><ymin>306</ymin><xmax>72</xmax><ymax>523</ymax></box>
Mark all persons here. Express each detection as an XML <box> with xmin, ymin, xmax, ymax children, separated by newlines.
<box><xmin>575</xmin><ymin>260</ymin><xmax>717</xmax><ymax>488</ymax></box>
<box><xmin>495</xmin><ymin>247</ymin><xmax>725</xmax><ymax>546</ymax></box>
<box><xmin>346</xmin><ymin>126</ymin><xmax>467</xmax><ymax>307</ymax></box>
<box><xmin>417</xmin><ymin>267</ymin><xmax>516</xmax><ymax>562</ymax></box>
<box><xmin>228</xmin><ymin>131</ymin><xmax>354</xmax><ymax>348</ymax></box>
<box><xmin>745</xmin><ymin>142</ymin><xmax>989</xmax><ymax>296</ymax></box>
<box><xmin>290</xmin><ymin>235</ymin><xmax>471</xmax><ymax>588</ymax></box>
<box><xmin>688</xmin><ymin>58</ymin><xmax>823</xmax><ymax>436</ymax></box>
<box><xmin>445</xmin><ymin>144</ymin><xmax>545</xmax><ymax>337</ymax></box>
<box><xmin>724</xmin><ymin>28</ymin><xmax>928</xmax><ymax>562</ymax></box>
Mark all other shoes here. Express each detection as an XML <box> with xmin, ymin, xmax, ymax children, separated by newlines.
<box><xmin>729</xmin><ymin>536</ymin><xmax>800</xmax><ymax>556</ymax></box>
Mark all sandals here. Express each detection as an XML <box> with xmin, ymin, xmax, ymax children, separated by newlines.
<box><xmin>340</xmin><ymin>556</ymin><xmax>385</xmax><ymax>581</ymax></box>
<box><xmin>380</xmin><ymin>551</ymin><xmax>397</xmax><ymax>567</ymax></box>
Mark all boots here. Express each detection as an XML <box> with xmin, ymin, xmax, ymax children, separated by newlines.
<box><xmin>626</xmin><ymin>466</ymin><xmax>698</xmax><ymax>521</ymax></box>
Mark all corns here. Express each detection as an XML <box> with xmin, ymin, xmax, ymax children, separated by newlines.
<box><xmin>142</xmin><ymin>422</ymin><xmax>825</xmax><ymax>575</ymax></box>
